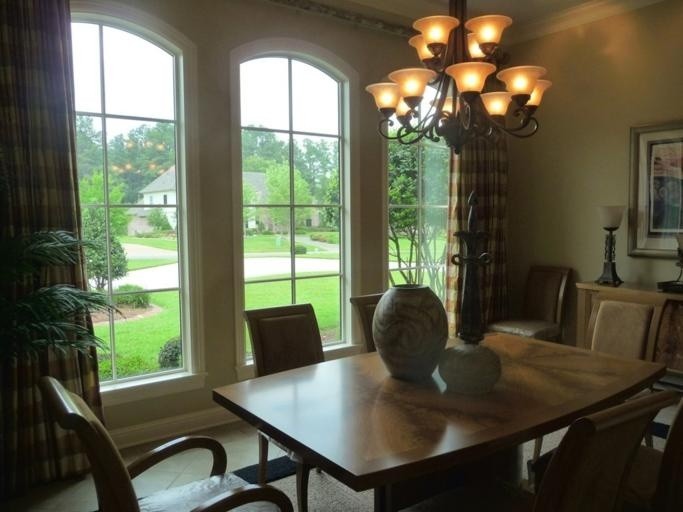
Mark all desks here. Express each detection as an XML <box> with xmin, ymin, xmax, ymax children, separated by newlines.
<box><xmin>210</xmin><ymin>329</ymin><xmax>669</xmax><ymax>509</ymax></box>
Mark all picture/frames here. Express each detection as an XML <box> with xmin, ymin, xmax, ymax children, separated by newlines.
<box><xmin>628</xmin><ymin>119</ymin><xmax>682</xmax><ymax>261</ymax></box>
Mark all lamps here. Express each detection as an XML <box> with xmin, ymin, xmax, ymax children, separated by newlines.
<box><xmin>595</xmin><ymin>204</ymin><xmax>624</xmax><ymax>286</ymax></box>
<box><xmin>365</xmin><ymin>0</ymin><xmax>552</xmax><ymax>156</ymax></box>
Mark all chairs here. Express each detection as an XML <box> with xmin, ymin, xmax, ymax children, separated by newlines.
<box><xmin>485</xmin><ymin>264</ymin><xmax>575</xmax><ymax>343</ymax></box>
<box><xmin>404</xmin><ymin>386</ymin><xmax>683</xmax><ymax>508</ymax></box>
<box><xmin>38</xmin><ymin>374</ymin><xmax>294</xmax><ymax>508</ymax></box>
<box><xmin>239</xmin><ymin>301</ymin><xmax>357</xmax><ymax>486</ymax></box>
<box><xmin>347</xmin><ymin>295</ymin><xmax>388</xmax><ymax>355</ymax></box>
<box><xmin>584</xmin><ymin>294</ymin><xmax>672</xmax><ymax>450</ymax></box>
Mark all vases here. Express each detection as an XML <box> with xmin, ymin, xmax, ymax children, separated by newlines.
<box><xmin>372</xmin><ymin>282</ymin><xmax>448</xmax><ymax>384</ymax></box>
